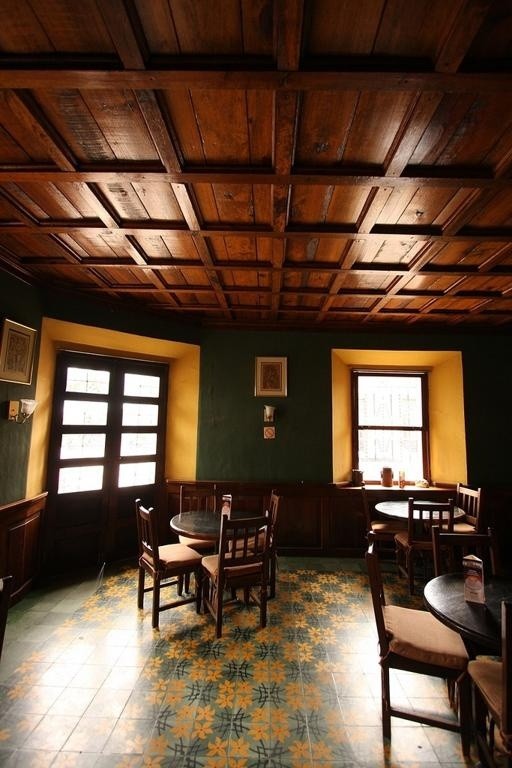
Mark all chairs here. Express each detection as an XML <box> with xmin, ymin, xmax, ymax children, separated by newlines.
<box><xmin>135</xmin><ymin>483</ymin><xmax>284</xmax><ymax>638</ymax></box>
<box><xmin>360</xmin><ymin>483</ymin><xmax>512</xmax><ymax>768</ymax></box>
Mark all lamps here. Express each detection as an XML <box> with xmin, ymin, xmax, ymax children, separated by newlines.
<box><xmin>263</xmin><ymin>405</ymin><xmax>274</xmax><ymax>422</ymax></box>
<box><xmin>7</xmin><ymin>398</ymin><xmax>38</xmax><ymax>424</ymax></box>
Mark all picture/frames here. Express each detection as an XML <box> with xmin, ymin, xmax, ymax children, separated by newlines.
<box><xmin>254</xmin><ymin>355</ymin><xmax>288</xmax><ymax>398</ymax></box>
<box><xmin>0</xmin><ymin>318</ymin><xmax>37</xmax><ymax>386</ymax></box>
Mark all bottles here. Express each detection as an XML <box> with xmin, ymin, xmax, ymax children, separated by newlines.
<box><xmin>398</xmin><ymin>469</ymin><xmax>406</xmax><ymax>488</ymax></box>
<box><xmin>380</xmin><ymin>468</ymin><xmax>395</xmax><ymax>487</ymax></box>
